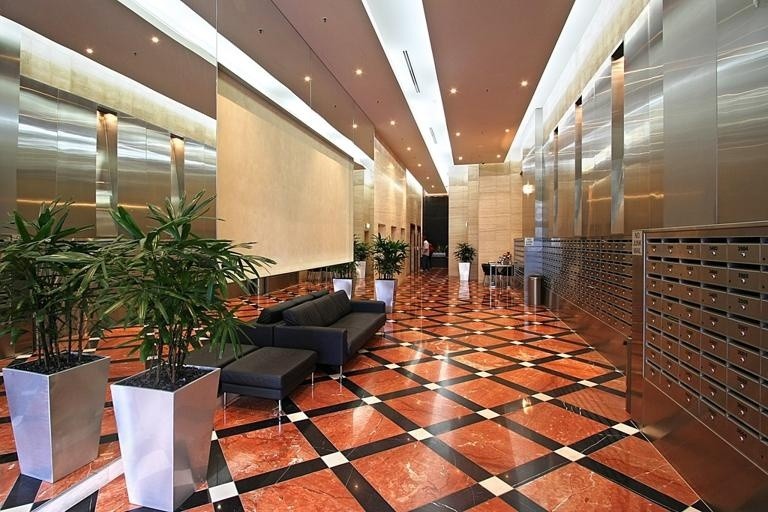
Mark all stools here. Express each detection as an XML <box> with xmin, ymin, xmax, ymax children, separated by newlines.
<box><xmin>176</xmin><ymin>343</ymin><xmax>316</xmax><ymax>415</ymax></box>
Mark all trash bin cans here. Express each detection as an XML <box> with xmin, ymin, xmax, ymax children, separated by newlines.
<box><xmin>528</xmin><ymin>274</ymin><xmax>544</xmax><ymax>305</ymax></box>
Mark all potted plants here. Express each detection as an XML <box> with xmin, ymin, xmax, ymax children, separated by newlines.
<box><xmin>76</xmin><ymin>187</ymin><xmax>277</xmax><ymax>512</ymax></box>
<box><xmin>452</xmin><ymin>242</ymin><xmax>478</xmax><ymax>281</ymax></box>
<box><xmin>1</xmin><ymin>195</ymin><xmax>126</xmax><ymax>484</ymax></box>
<box><xmin>326</xmin><ymin>233</ymin><xmax>412</xmax><ymax>314</ymax></box>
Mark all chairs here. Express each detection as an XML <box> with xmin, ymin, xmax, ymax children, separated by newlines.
<box><xmin>481</xmin><ymin>263</ymin><xmax>514</xmax><ymax>286</ymax></box>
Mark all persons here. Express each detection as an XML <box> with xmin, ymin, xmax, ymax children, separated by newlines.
<box><xmin>428</xmin><ymin>242</ymin><xmax>434</xmax><ymax>269</ymax></box>
<box><xmin>423</xmin><ymin>237</ymin><xmax>431</xmax><ymax>274</ymax></box>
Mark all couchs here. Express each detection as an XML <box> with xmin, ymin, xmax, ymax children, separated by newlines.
<box><xmin>246</xmin><ymin>288</ymin><xmax>386</xmax><ymax>377</ymax></box>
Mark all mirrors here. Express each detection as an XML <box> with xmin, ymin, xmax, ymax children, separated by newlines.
<box><xmin>0</xmin><ymin>0</ymin><xmax>216</xmax><ymax>512</ymax></box>
